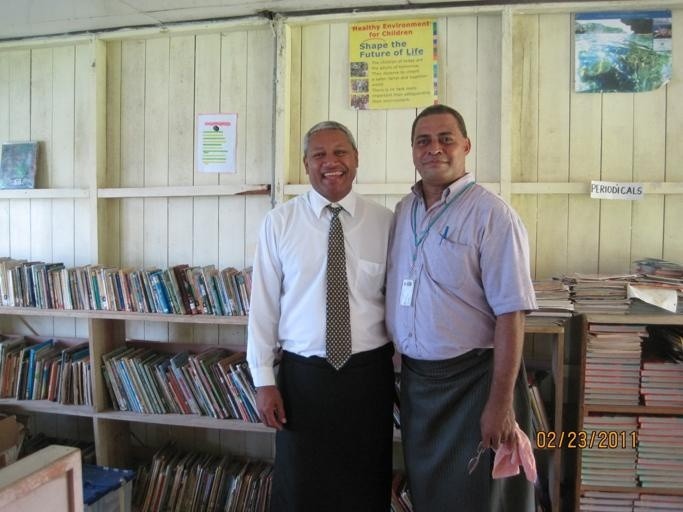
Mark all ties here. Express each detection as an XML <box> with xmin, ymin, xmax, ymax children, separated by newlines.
<box><xmin>325</xmin><ymin>206</ymin><xmax>351</xmax><ymax>369</ymax></box>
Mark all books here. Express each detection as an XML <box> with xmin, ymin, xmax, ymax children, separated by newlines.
<box><xmin>99</xmin><ymin>347</ymin><xmax>265</xmax><ymax>423</ymax></box>
<box><xmin>392</xmin><ymin>371</ymin><xmax>404</xmax><ymax>429</ymax></box>
<box><xmin>581</xmin><ymin>415</ymin><xmax>682</xmax><ymax>488</ymax></box>
<box><xmin>22</xmin><ymin>432</ymin><xmax>96</xmax><ymax>467</ymax></box>
<box><xmin>0</xmin><ymin>258</ymin><xmax>253</xmax><ymax>317</ymax></box>
<box><xmin>583</xmin><ymin>325</ymin><xmax>682</xmax><ymax>407</ymax></box>
<box><xmin>132</xmin><ymin>442</ymin><xmax>281</xmax><ymax>511</ymax></box>
<box><xmin>511</xmin><ymin>372</ymin><xmax>549</xmax><ymax>438</ymax></box>
<box><xmin>389</xmin><ymin>468</ymin><xmax>414</xmax><ymax>512</ymax></box>
<box><xmin>525</xmin><ymin>260</ymin><xmax>682</xmax><ymax>317</ymax></box>
<box><xmin>0</xmin><ymin>335</ymin><xmax>92</xmax><ymax>404</ymax></box>
<box><xmin>578</xmin><ymin>489</ymin><xmax>683</xmax><ymax>512</ymax></box>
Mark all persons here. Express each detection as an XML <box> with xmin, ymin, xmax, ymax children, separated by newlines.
<box><xmin>245</xmin><ymin>120</ymin><xmax>396</xmax><ymax>512</ymax></box>
<box><xmin>384</xmin><ymin>103</ymin><xmax>536</xmax><ymax>511</ymax></box>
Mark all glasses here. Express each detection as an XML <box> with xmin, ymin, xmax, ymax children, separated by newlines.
<box><xmin>467</xmin><ymin>441</ymin><xmax>496</xmax><ymax>473</ymax></box>
